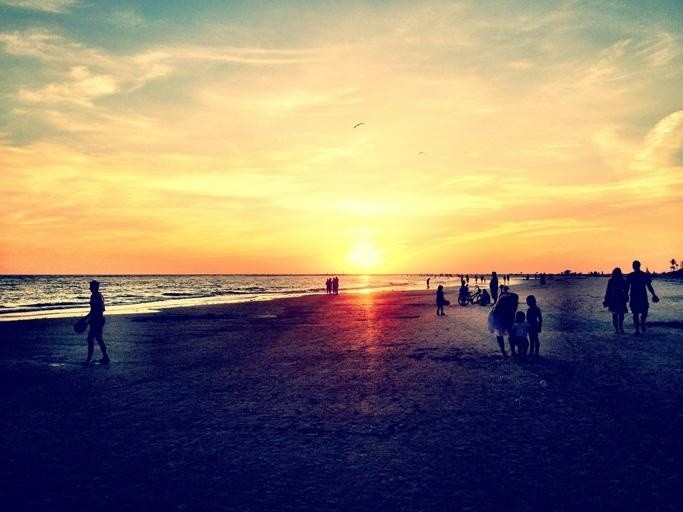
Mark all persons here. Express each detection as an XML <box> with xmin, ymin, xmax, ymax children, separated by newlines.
<box><xmin>74</xmin><ymin>280</ymin><xmax>111</xmax><ymax>366</ymax></box>
<box><xmin>425</xmin><ymin>271</ymin><xmax>601</xmax><ymax>357</ymax></box>
<box><xmin>603</xmin><ymin>268</ymin><xmax>630</xmax><ymax>335</ymax></box>
<box><xmin>626</xmin><ymin>261</ymin><xmax>656</xmax><ymax>335</ymax></box>
<box><xmin>326</xmin><ymin>277</ymin><xmax>339</xmax><ymax>295</ymax></box>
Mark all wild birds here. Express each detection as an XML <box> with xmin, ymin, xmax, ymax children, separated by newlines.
<box><xmin>353</xmin><ymin>123</ymin><xmax>363</xmax><ymax>128</ymax></box>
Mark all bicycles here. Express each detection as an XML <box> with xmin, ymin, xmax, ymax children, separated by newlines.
<box><xmin>458</xmin><ymin>287</ymin><xmax>483</xmax><ymax>306</ymax></box>
